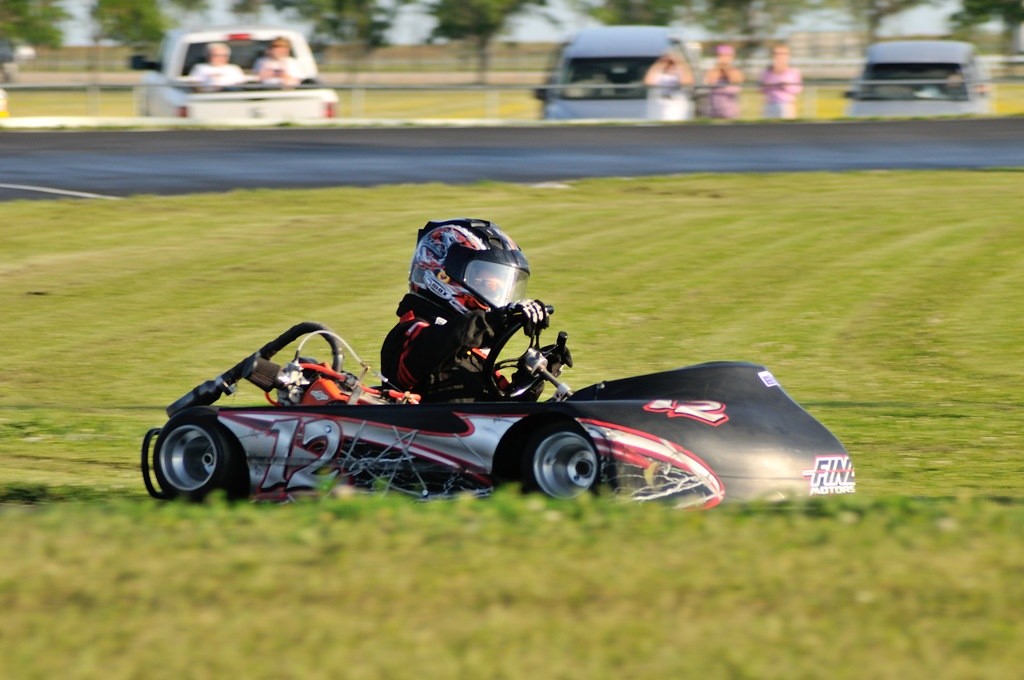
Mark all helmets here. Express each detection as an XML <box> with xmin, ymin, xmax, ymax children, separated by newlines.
<box><xmin>408</xmin><ymin>217</ymin><xmax>530</xmax><ymax>323</ymax></box>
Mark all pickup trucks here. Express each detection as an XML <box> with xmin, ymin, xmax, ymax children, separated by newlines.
<box><xmin>128</xmin><ymin>26</ymin><xmax>341</xmax><ymax>120</ymax></box>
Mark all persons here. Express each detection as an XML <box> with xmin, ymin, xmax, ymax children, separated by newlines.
<box><xmin>188</xmin><ymin>43</ymin><xmax>246</xmax><ymax>94</ymax></box>
<box><xmin>642</xmin><ymin>38</ymin><xmax>803</xmax><ymax>119</ymax></box>
<box><xmin>252</xmin><ymin>36</ymin><xmax>303</xmax><ymax>87</ymax></box>
<box><xmin>382</xmin><ymin>219</ymin><xmax>573</xmax><ymax>404</ymax></box>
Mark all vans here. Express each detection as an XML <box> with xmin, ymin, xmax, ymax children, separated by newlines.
<box><xmin>529</xmin><ymin>25</ymin><xmax>695</xmax><ymax>126</ymax></box>
<box><xmin>846</xmin><ymin>36</ymin><xmax>997</xmax><ymax>119</ymax></box>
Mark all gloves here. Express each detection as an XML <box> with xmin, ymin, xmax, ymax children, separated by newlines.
<box><xmin>515</xmin><ymin>344</ymin><xmax>573</xmax><ymax>385</ymax></box>
<box><xmin>485</xmin><ymin>298</ymin><xmax>549</xmax><ymax>337</ymax></box>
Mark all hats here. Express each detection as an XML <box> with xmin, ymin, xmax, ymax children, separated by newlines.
<box><xmin>717</xmin><ymin>45</ymin><xmax>734</xmax><ymax>55</ymax></box>
